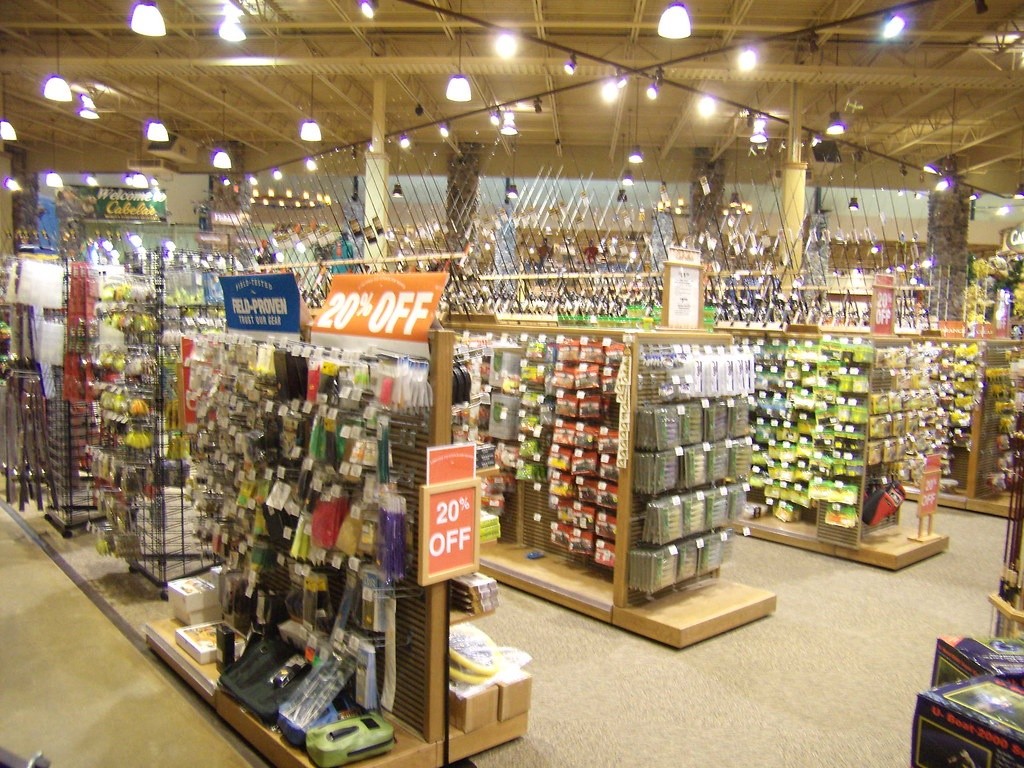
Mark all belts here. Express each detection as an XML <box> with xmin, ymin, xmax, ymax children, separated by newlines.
<box><xmin>0</xmin><ymin>372</ymin><xmax>59</xmax><ymax>511</ymax></box>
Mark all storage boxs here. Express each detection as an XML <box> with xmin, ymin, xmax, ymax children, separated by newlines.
<box><xmin>167</xmin><ymin>565</ymin><xmax>244</xmax><ymax>667</ymax></box>
<box><xmin>910</xmin><ymin>638</ymin><xmax>1024</xmax><ymax>768</ymax></box>
<box><xmin>480</xmin><ymin>512</ymin><xmax>502</xmax><ymax>543</ymax></box>
<box><xmin>447</xmin><ymin>670</ymin><xmax>533</xmax><ymax>734</ymax></box>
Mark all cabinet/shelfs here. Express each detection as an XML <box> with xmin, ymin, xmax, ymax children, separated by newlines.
<box><xmin>456</xmin><ymin>321</ymin><xmax>1023</xmax><ymax>649</ymax></box>
<box><xmin>141</xmin><ymin>321</ymin><xmax>534</xmax><ymax>768</ymax></box>
<box><xmin>0</xmin><ymin>250</ymin><xmax>229</xmax><ymax>599</ymax></box>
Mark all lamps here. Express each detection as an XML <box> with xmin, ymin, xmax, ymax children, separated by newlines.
<box><xmin>656</xmin><ymin>0</ymin><xmax>692</xmax><ymax>39</ymax></box>
<box><xmin>216</xmin><ymin>4</ymin><xmax>247</xmax><ymax>41</ymax></box>
<box><xmin>879</xmin><ymin>10</ymin><xmax>905</xmax><ymax>39</ymax></box>
<box><xmin>489</xmin><ymin>106</ymin><xmax>502</xmax><ymax>125</ymax></box>
<box><xmin>848</xmin><ymin>163</ymin><xmax>859</xmax><ymax>211</ymax></box>
<box><xmin>398</xmin><ymin>131</ymin><xmax>412</xmax><ymax>149</ymax></box>
<box><xmin>86</xmin><ymin>172</ymin><xmax>100</xmax><ymax>187</ymax></box>
<box><xmin>124</xmin><ymin>172</ymin><xmax>135</xmax><ymax>185</ymax></box>
<box><xmin>564</xmin><ymin>53</ymin><xmax>579</xmax><ymax>78</ymax></box>
<box><xmin>507</xmin><ymin>152</ymin><xmax>519</xmax><ymax>198</ymax></box>
<box><xmin>499</xmin><ymin>104</ymin><xmax>518</xmax><ymax>136</ymax></box>
<box><xmin>439</xmin><ymin>120</ymin><xmax>450</xmax><ymax>138</ymax></box>
<box><xmin>211</xmin><ymin>89</ymin><xmax>233</xmax><ymax>169</ymax></box>
<box><xmin>356</xmin><ymin>1</ymin><xmax>379</xmax><ymax>18</ymax></box>
<box><xmin>645</xmin><ymin>67</ymin><xmax>665</xmax><ymax>100</ymax></box>
<box><xmin>0</xmin><ymin>71</ymin><xmax>18</xmax><ymax>140</ymax></box>
<box><xmin>393</xmin><ymin>149</ymin><xmax>404</xmax><ymax>199</ymax></box>
<box><xmin>299</xmin><ymin>72</ymin><xmax>324</xmax><ymax>142</ymax></box>
<box><xmin>806</xmin><ymin>31</ymin><xmax>821</xmax><ymax>57</ymax></box>
<box><xmin>616</xmin><ymin>189</ymin><xmax>627</xmax><ymax>202</ymax></box>
<box><xmin>445</xmin><ymin>0</ymin><xmax>473</xmax><ymax>103</ymax></box>
<box><xmin>271</xmin><ymin>166</ymin><xmax>282</xmax><ymax>180</ymax></box>
<box><xmin>616</xmin><ymin>68</ymin><xmax>628</xmax><ymax>89</ymax></box>
<box><xmin>43</xmin><ymin>1</ymin><xmax>74</xmax><ymax>102</ymax></box>
<box><xmin>130</xmin><ymin>0</ymin><xmax>167</xmax><ymax>36</ymax></box>
<box><xmin>304</xmin><ymin>156</ymin><xmax>317</xmax><ymax>172</ymax></box>
<box><xmin>627</xmin><ymin>77</ymin><xmax>645</xmax><ymax>164</ymax></box>
<box><xmin>944</xmin><ymin>89</ymin><xmax>958</xmax><ymax>175</ymax></box>
<box><xmin>826</xmin><ymin>34</ymin><xmax>847</xmax><ymax>136</ymax></box>
<box><xmin>730</xmin><ymin>137</ymin><xmax>742</xmax><ymax>207</ymax></box>
<box><xmin>45</xmin><ymin>119</ymin><xmax>64</xmax><ymax>188</ymax></box>
<box><xmin>973</xmin><ymin>0</ymin><xmax>990</xmax><ymax>15</ymax></box>
<box><xmin>897</xmin><ymin>162</ymin><xmax>907</xmax><ymax>176</ymax></box>
<box><xmin>750</xmin><ymin>91</ymin><xmax>768</xmax><ymax>144</ymax></box>
<box><xmin>1013</xmin><ymin>183</ymin><xmax>1024</xmax><ymax>199</ymax></box>
<box><xmin>622</xmin><ymin>108</ymin><xmax>635</xmax><ymax>186</ymax></box>
<box><xmin>146</xmin><ymin>73</ymin><xmax>170</xmax><ymax>142</ymax></box>
